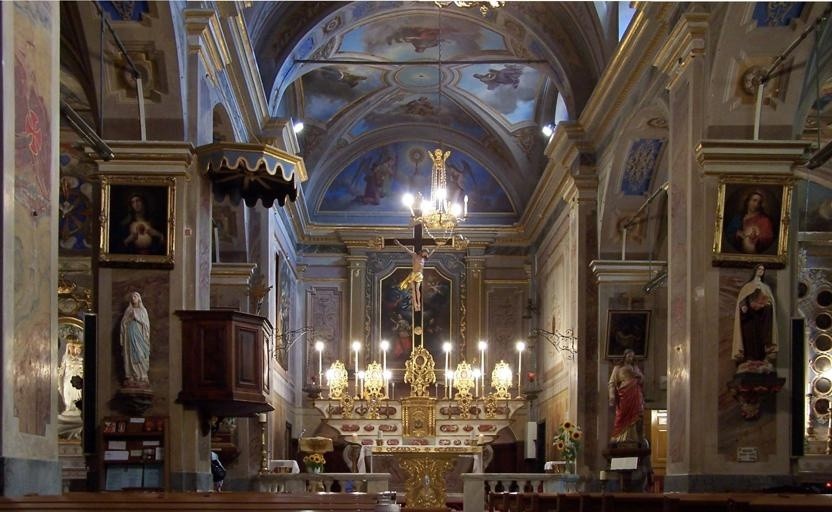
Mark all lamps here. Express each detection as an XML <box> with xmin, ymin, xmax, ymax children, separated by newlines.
<box><xmin>407</xmin><ymin>1</ymin><xmax>470</xmax><ymax>243</ymax></box>
<box><xmin>542</xmin><ymin>123</ymin><xmax>557</xmax><ymax>137</ymax></box>
<box><xmin>291</xmin><ymin>119</ymin><xmax>309</xmax><ymax>138</ymax></box>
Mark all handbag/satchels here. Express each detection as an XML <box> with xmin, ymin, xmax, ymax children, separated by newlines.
<box><xmin>210</xmin><ymin>460</ymin><xmax>225</xmax><ymax>482</ymax></box>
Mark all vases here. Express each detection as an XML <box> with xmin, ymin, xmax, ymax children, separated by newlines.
<box><xmin>562</xmin><ymin>454</ymin><xmax>573</xmax><ymax>475</ymax></box>
<box><xmin>305</xmin><ymin>467</ymin><xmax>321</xmax><ymax>491</ymax></box>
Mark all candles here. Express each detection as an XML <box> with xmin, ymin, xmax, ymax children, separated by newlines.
<box><xmin>469</xmin><ymin>431</ymin><xmax>476</xmax><ymax>441</ymax></box>
<box><xmin>477</xmin><ymin>339</ymin><xmax>490</xmax><ymax>374</ymax></box>
<box><xmin>314</xmin><ymin>339</ymin><xmax>326</xmax><ymax>375</ymax></box>
<box><xmin>351</xmin><ymin>339</ymin><xmax>361</xmax><ymax>373</ymax></box>
<box><xmin>380</xmin><ymin>339</ymin><xmax>390</xmax><ymax>379</ymax></box>
<box><xmin>441</xmin><ymin>341</ymin><xmax>452</xmax><ymax>375</ymax></box>
<box><xmin>478</xmin><ymin>433</ymin><xmax>484</xmax><ymax>444</ymax></box>
<box><xmin>352</xmin><ymin>433</ymin><xmax>358</xmax><ymax>443</ymax></box>
<box><xmin>378</xmin><ymin>430</ymin><xmax>383</xmax><ymax>439</ymax></box>
<box><xmin>515</xmin><ymin>340</ymin><xmax>528</xmax><ymax>375</ymax></box>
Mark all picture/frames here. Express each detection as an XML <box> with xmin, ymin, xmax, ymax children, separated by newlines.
<box><xmin>94</xmin><ymin>176</ymin><xmax>177</xmax><ymax>264</ymax></box>
<box><xmin>711</xmin><ymin>176</ymin><xmax>792</xmax><ymax>264</ymax></box>
<box><xmin>602</xmin><ymin>307</ymin><xmax>653</xmax><ymax>361</ymax></box>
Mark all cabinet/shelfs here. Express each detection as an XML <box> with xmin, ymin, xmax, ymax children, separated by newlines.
<box><xmin>102</xmin><ymin>431</ymin><xmax>164</xmax><ymax>466</ymax></box>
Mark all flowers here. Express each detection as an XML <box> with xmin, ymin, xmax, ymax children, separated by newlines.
<box><xmin>550</xmin><ymin>420</ymin><xmax>584</xmax><ymax>463</ymax></box>
<box><xmin>302</xmin><ymin>453</ymin><xmax>327</xmax><ymax>469</ymax></box>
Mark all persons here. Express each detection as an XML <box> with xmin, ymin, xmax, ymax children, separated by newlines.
<box><xmin>607</xmin><ymin>348</ymin><xmax>648</xmax><ymax>445</ymax></box>
<box><xmin>391</xmin><ymin>238</ymin><xmax>442</xmax><ymax>313</ymax></box>
<box><xmin>56</xmin><ymin>342</ymin><xmax>84</xmax><ymax>411</ymax></box>
<box><xmin>730</xmin><ymin>260</ymin><xmax>779</xmax><ymax>365</ymax></box>
<box><xmin>732</xmin><ymin>189</ymin><xmax>775</xmax><ymax>255</ymax></box>
<box><xmin>385</xmin><ymin>277</ymin><xmax>449</xmax><ymax>364</ymax></box>
<box><xmin>117</xmin><ymin>291</ymin><xmax>154</xmax><ymax>386</ymax></box>
<box><xmin>120</xmin><ymin>193</ymin><xmax>166</xmax><ymax>255</ymax></box>
